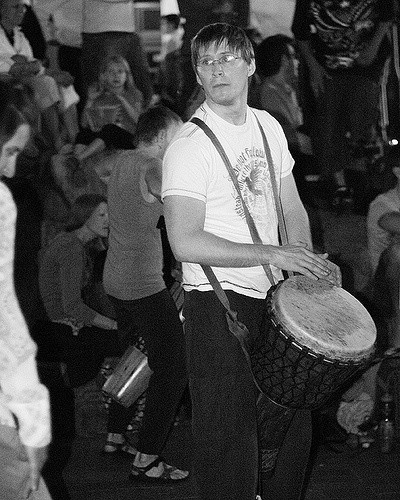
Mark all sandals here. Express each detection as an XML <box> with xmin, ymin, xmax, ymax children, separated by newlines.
<box><xmin>102</xmin><ymin>439</ymin><xmax>137</xmax><ymax>459</ymax></box>
<box><xmin>128</xmin><ymin>456</ymin><xmax>190</xmax><ymax>484</ymax></box>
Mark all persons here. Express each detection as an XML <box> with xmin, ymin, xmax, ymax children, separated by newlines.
<box><xmin>0</xmin><ymin>0</ymin><xmax>400</xmax><ymax>484</ymax></box>
<box><xmin>0</xmin><ymin>104</ymin><xmax>54</xmax><ymax>500</ymax></box>
<box><xmin>160</xmin><ymin>23</ymin><xmax>342</xmax><ymax>500</ymax></box>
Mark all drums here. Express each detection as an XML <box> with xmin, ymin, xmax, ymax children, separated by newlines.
<box><xmin>249</xmin><ymin>274</ymin><xmax>378</xmax><ymax>488</ymax></box>
<box><xmin>101</xmin><ymin>268</ymin><xmax>186</xmax><ymax>409</ymax></box>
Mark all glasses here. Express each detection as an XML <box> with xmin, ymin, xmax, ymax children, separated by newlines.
<box><xmin>195</xmin><ymin>55</ymin><xmax>242</xmax><ymax>66</ymax></box>
<box><xmin>283</xmin><ymin>54</ymin><xmax>300</xmax><ymax>60</ymax></box>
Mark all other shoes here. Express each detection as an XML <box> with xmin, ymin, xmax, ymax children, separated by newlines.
<box><xmin>63</xmin><ymin>154</ymin><xmax>78</xmax><ymax>170</ymax></box>
<box><xmin>331</xmin><ymin>188</ymin><xmax>352</xmax><ymax>208</ymax></box>
